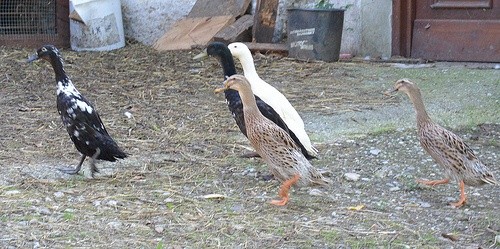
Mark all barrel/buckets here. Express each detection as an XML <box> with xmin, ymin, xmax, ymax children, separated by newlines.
<box><xmin>68</xmin><ymin>0</ymin><xmax>126</xmax><ymax>52</ymax></box>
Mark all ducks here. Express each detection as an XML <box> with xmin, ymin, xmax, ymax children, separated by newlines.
<box><xmin>386</xmin><ymin>78</ymin><xmax>495</xmax><ymax>207</ymax></box>
<box><xmin>192</xmin><ymin>43</ymin><xmax>320</xmax><ymax>182</ymax></box>
<box><xmin>26</xmin><ymin>45</ymin><xmax>128</xmax><ymax>178</ymax></box>
<box><xmin>214</xmin><ymin>74</ymin><xmax>322</xmax><ymax>206</ymax></box>
<box><xmin>227</xmin><ymin>42</ymin><xmax>320</xmax><ymax>157</ymax></box>
